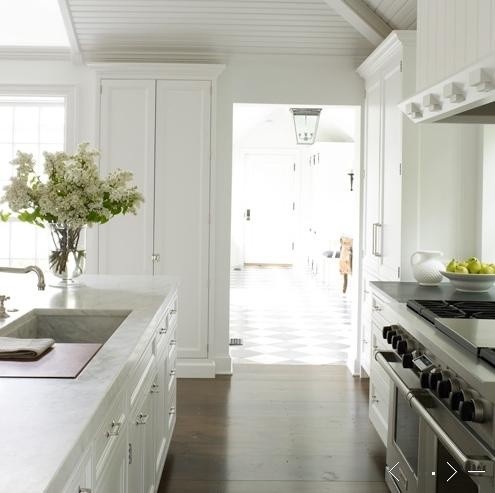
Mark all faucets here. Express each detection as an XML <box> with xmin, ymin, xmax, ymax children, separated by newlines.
<box><xmin>0</xmin><ymin>263</ymin><xmax>46</xmax><ymax>294</ymax></box>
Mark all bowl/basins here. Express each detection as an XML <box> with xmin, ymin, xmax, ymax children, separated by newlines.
<box><xmin>438</xmin><ymin>269</ymin><xmax>495</xmax><ymax>293</ymax></box>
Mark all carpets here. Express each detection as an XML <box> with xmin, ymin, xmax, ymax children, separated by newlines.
<box><xmin>229</xmin><ymin>337</ymin><xmax>243</xmax><ymax>345</ymax></box>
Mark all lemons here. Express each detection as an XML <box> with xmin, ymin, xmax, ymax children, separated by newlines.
<box><xmin>447</xmin><ymin>256</ymin><xmax>495</xmax><ymax>274</ymax></box>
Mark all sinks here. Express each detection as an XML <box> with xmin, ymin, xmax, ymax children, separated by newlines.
<box><xmin>0</xmin><ymin>307</ymin><xmax>134</xmax><ymax>349</ymax></box>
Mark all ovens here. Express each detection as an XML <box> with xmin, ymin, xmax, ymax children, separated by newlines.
<box><xmin>369</xmin><ymin>345</ymin><xmax>495</xmax><ymax>492</ymax></box>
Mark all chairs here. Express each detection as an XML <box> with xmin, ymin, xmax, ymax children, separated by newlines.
<box><xmin>340</xmin><ymin>238</ymin><xmax>352</xmax><ymax>292</ymax></box>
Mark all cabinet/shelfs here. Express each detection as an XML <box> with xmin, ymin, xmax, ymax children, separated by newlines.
<box><xmin>128</xmin><ymin>313</ymin><xmax>171</xmax><ymax>493</ymax></box>
<box><xmin>368</xmin><ymin>281</ymin><xmax>404</xmax><ymax>448</ymax></box>
<box><xmin>65</xmin><ymin>61</ymin><xmax>233</xmax><ymax>379</ymax></box>
<box><xmin>167</xmin><ymin>288</ymin><xmax>178</xmax><ymax>452</ymax></box>
<box><xmin>356</xmin><ymin>30</ymin><xmax>483</xmax><ymax>377</ymax></box>
<box><xmin>62</xmin><ymin>383</ymin><xmax>128</xmax><ymax>493</ymax></box>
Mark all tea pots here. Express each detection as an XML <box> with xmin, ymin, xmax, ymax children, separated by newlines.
<box><xmin>410</xmin><ymin>249</ymin><xmax>447</xmax><ymax>286</ymax></box>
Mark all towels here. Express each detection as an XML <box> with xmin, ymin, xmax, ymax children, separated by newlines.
<box><xmin>0</xmin><ymin>336</ymin><xmax>55</xmax><ymax>358</ymax></box>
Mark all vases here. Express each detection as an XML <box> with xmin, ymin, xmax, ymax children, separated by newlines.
<box><xmin>46</xmin><ymin>223</ymin><xmax>85</xmax><ymax>283</ymax></box>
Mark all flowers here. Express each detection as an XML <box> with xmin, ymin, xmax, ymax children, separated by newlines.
<box><xmin>0</xmin><ymin>140</ymin><xmax>145</xmax><ymax>274</ymax></box>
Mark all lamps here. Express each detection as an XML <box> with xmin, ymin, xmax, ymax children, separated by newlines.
<box><xmin>290</xmin><ymin>107</ymin><xmax>323</xmax><ymax>145</ymax></box>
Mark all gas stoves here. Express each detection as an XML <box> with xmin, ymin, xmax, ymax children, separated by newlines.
<box><xmin>382</xmin><ymin>293</ymin><xmax>495</xmax><ymax>448</ymax></box>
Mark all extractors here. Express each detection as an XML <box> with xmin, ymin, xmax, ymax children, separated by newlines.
<box><xmin>397</xmin><ymin>1</ymin><xmax>495</xmax><ymax>125</ymax></box>
